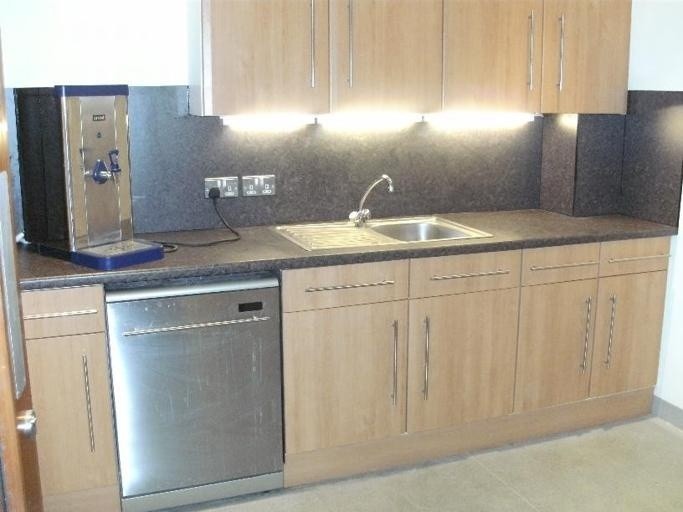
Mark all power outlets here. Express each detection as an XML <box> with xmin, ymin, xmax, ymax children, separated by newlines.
<box><xmin>198</xmin><ymin>175</ymin><xmax>279</xmax><ymax>201</ymax></box>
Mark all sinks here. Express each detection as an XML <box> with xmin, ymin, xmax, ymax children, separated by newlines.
<box><xmin>368</xmin><ymin>216</ymin><xmax>493</xmax><ymax>243</ymax></box>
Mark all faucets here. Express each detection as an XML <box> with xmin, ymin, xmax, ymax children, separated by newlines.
<box><xmin>93</xmin><ymin>150</ymin><xmax>122</xmax><ymax>189</ymax></box>
<box><xmin>347</xmin><ymin>174</ymin><xmax>394</xmax><ymax>226</ymax></box>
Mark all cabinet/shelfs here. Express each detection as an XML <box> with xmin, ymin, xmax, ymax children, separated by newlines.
<box><xmin>19</xmin><ymin>283</ymin><xmax>116</xmax><ymax>511</ymax></box>
<box><xmin>442</xmin><ymin>1</ymin><xmax>631</xmax><ymax>114</ymax></box>
<box><xmin>191</xmin><ymin>1</ymin><xmax>441</xmax><ymax>115</ymax></box>
